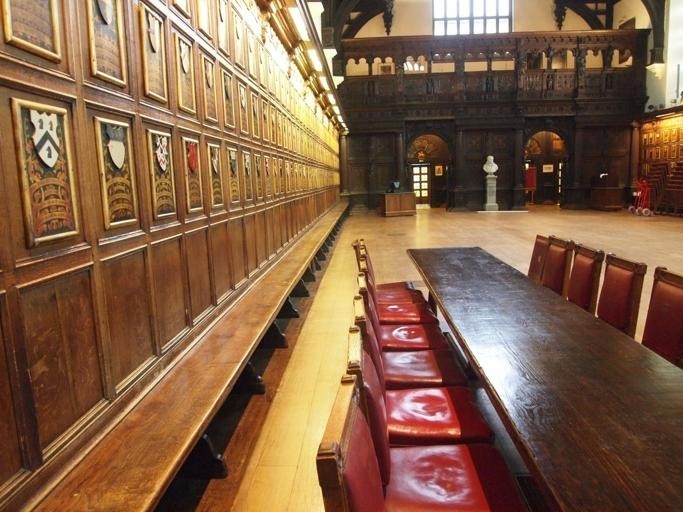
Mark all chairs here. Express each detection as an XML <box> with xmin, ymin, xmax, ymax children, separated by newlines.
<box><xmin>541</xmin><ymin>235</ymin><xmax>575</xmax><ymax>298</ymax></box>
<box><xmin>357</xmin><ymin>271</ymin><xmax>453</xmax><ymax>351</ymax></box>
<box><xmin>351</xmin><ymin>238</ymin><xmax>429</xmax><ymax>304</ymax></box>
<box><xmin>597</xmin><ymin>253</ymin><xmax>647</xmax><ymax>339</ymax></box>
<box><xmin>316</xmin><ymin>373</ymin><xmax>536</xmax><ymax>512</ymax></box>
<box><xmin>353</xmin><ymin>293</ymin><xmax>469</xmax><ymax>389</ymax></box>
<box><xmin>528</xmin><ymin>234</ymin><xmax>555</xmax><ymax>282</ymax></box>
<box><xmin>346</xmin><ymin>324</ymin><xmax>497</xmax><ymax>447</ymax></box>
<box><xmin>641</xmin><ymin>265</ymin><xmax>683</xmax><ymax>372</ymax></box>
<box><xmin>568</xmin><ymin>242</ymin><xmax>605</xmax><ymax>315</ymax></box>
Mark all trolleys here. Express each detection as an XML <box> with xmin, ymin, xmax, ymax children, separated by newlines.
<box><xmin>629</xmin><ymin>178</ymin><xmax>655</xmax><ymax>218</ymax></box>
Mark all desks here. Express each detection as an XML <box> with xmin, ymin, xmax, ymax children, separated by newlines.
<box><xmin>406</xmin><ymin>245</ymin><xmax>683</xmax><ymax>512</ymax></box>
